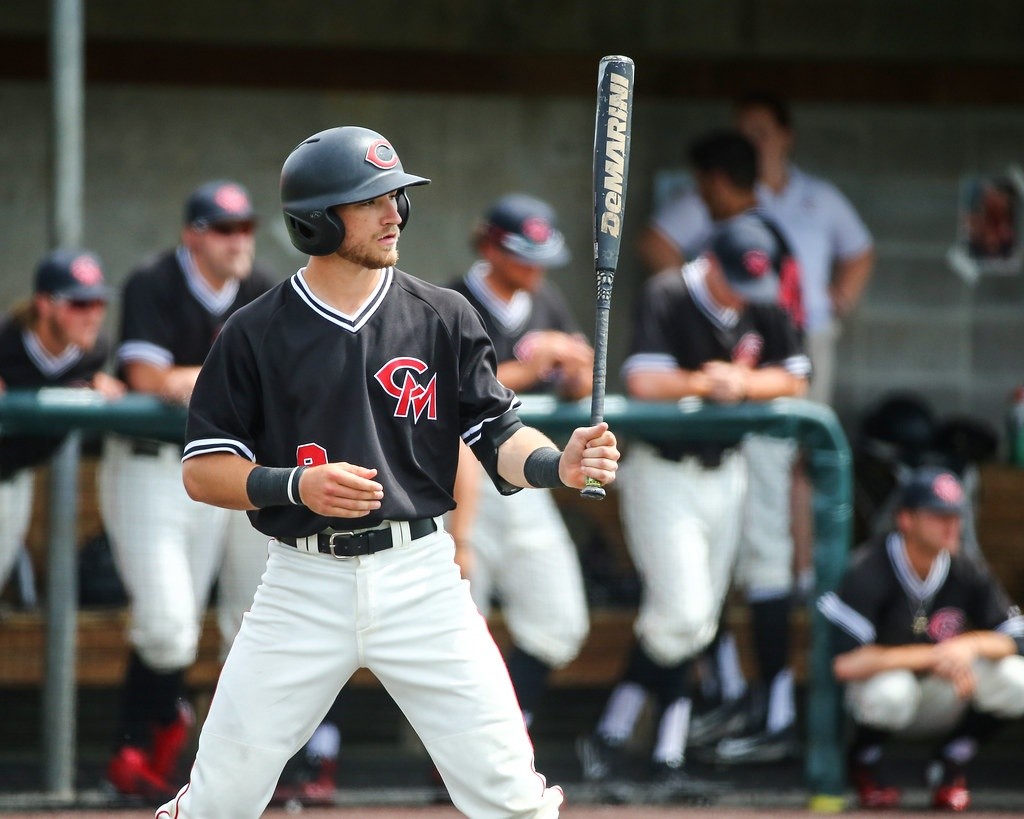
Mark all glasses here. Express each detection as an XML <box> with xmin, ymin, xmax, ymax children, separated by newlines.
<box><xmin>207</xmin><ymin>219</ymin><xmax>255</xmax><ymax>234</ymax></box>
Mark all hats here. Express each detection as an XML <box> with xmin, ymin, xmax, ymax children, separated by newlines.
<box><xmin>35</xmin><ymin>248</ymin><xmax>121</xmax><ymax>299</ymax></box>
<box><xmin>715</xmin><ymin>215</ymin><xmax>780</xmax><ymax>301</ymax></box>
<box><xmin>903</xmin><ymin>465</ymin><xmax>970</xmax><ymax>514</ymax></box>
<box><xmin>482</xmin><ymin>194</ymin><xmax>564</xmax><ymax>259</ymax></box>
<box><xmin>184</xmin><ymin>180</ymin><xmax>262</xmax><ymax>226</ymax></box>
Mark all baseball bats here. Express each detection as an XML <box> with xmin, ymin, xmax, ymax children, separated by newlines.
<box><xmin>580</xmin><ymin>55</ymin><xmax>634</xmax><ymax>501</ymax></box>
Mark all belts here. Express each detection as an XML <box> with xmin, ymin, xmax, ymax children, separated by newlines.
<box><xmin>276</xmin><ymin>518</ymin><xmax>437</xmax><ymax>560</ymax></box>
<box><xmin>130</xmin><ymin>437</ymin><xmax>161</xmax><ymax>457</ymax></box>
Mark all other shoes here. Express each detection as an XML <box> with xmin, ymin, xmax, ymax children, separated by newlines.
<box><xmin>929</xmin><ymin>761</ymin><xmax>972</xmax><ymax>812</ymax></box>
<box><xmin>646</xmin><ymin>765</ymin><xmax>717</xmax><ymax>807</ymax></box>
<box><xmin>273</xmin><ymin>765</ymin><xmax>336</xmax><ymax>804</ymax></box>
<box><xmin>576</xmin><ymin>736</ymin><xmax>633</xmax><ymax>805</ymax></box>
<box><xmin>102</xmin><ymin>746</ymin><xmax>179</xmax><ymax>805</ymax></box>
<box><xmin>151</xmin><ymin>696</ymin><xmax>197</xmax><ymax>775</ymax></box>
<box><xmin>847</xmin><ymin>750</ymin><xmax>898</xmax><ymax>809</ymax></box>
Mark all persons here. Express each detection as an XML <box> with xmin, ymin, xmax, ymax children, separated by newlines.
<box><xmin>96</xmin><ymin>177</ymin><xmax>342</xmax><ymax>804</ymax></box>
<box><xmin>0</xmin><ymin>242</ymin><xmax>132</xmax><ymax>605</ymax></box>
<box><xmin>432</xmin><ymin>193</ymin><xmax>597</xmax><ymax>804</ymax></box>
<box><xmin>155</xmin><ymin>125</ymin><xmax>620</xmax><ymax>819</ymax></box>
<box><xmin>573</xmin><ymin>99</ymin><xmax>874</xmax><ymax>802</ymax></box>
<box><xmin>816</xmin><ymin>464</ymin><xmax>1024</xmax><ymax>811</ymax></box>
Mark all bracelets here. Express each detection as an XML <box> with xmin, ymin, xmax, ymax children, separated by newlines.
<box><xmin>246</xmin><ymin>466</ymin><xmax>306</xmax><ymax>507</ymax></box>
<box><xmin>524</xmin><ymin>447</ymin><xmax>567</xmax><ymax>489</ymax></box>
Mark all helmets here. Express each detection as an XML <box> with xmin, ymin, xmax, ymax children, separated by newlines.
<box><xmin>279</xmin><ymin>126</ymin><xmax>432</xmax><ymax>256</ymax></box>
